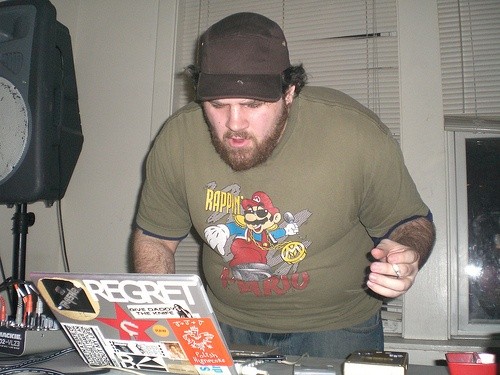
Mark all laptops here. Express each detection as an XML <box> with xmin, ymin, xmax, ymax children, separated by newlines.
<box><xmin>32</xmin><ymin>268</ymin><xmax>279</xmax><ymax>372</ymax></box>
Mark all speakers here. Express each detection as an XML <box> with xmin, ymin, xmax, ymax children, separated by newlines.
<box><xmin>0</xmin><ymin>1</ymin><xmax>84</xmax><ymax>205</ymax></box>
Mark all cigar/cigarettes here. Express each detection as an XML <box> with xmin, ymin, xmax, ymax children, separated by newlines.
<box><xmin>389</xmin><ymin>261</ymin><xmax>403</xmax><ymax>277</ymax></box>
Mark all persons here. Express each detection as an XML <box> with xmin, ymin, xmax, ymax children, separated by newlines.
<box><xmin>132</xmin><ymin>13</ymin><xmax>438</xmax><ymax>362</ymax></box>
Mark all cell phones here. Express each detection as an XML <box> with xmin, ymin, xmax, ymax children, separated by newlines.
<box><xmin>233</xmin><ymin>354</ymin><xmax>285</xmax><ymax>363</ymax></box>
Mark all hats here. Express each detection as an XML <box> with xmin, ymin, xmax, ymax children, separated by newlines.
<box><xmin>196</xmin><ymin>11</ymin><xmax>293</xmax><ymax>102</ymax></box>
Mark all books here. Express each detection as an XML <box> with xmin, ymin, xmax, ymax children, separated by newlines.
<box><xmin>341</xmin><ymin>350</ymin><xmax>410</xmax><ymax>375</ymax></box>
